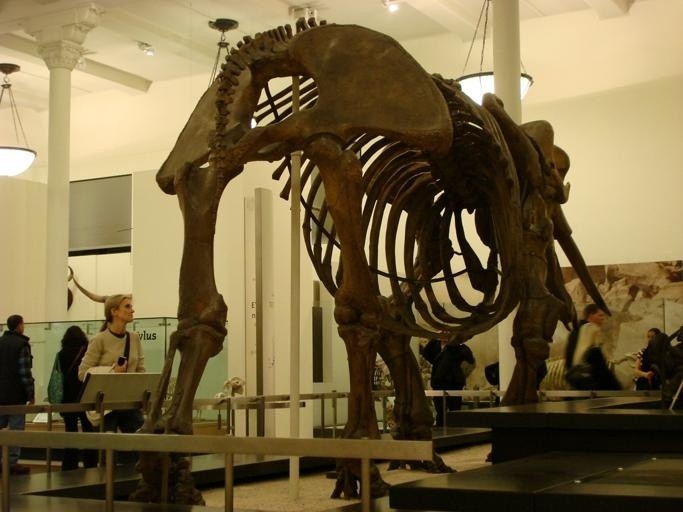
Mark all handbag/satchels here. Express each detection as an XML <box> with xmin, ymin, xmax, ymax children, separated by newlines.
<box><xmin>48</xmin><ymin>370</ymin><xmax>64</xmax><ymax>404</ymax></box>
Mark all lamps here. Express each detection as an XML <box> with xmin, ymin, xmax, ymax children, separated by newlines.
<box><xmin>452</xmin><ymin>2</ymin><xmax>533</xmax><ymax>106</ymax></box>
<box><xmin>1</xmin><ymin>62</ymin><xmax>36</xmax><ymax>177</ymax></box>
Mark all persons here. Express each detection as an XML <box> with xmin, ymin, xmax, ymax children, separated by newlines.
<box><xmin>0</xmin><ymin>313</ymin><xmax>34</xmax><ymax>474</ymax></box>
<box><xmin>46</xmin><ymin>325</ymin><xmax>99</xmax><ymax>471</ymax></box>
<box><xmin>424</xmin><ymin>326</ymin><xmax>476</xmax><ymax>427</ymax></box>
<box><xmin>562</xmin><ymin>303</ymin><xmax>604</xmax><ymax>389</ymax></box>
<box><xmin>75</xmin><ymin>293</ymin><xmax>146</xmax><ymax>467</ymax></box>
<box><xmin>633</xmin><ymin>327</ymin><xmax>662</xmax><ymax>390</ymax></box>
